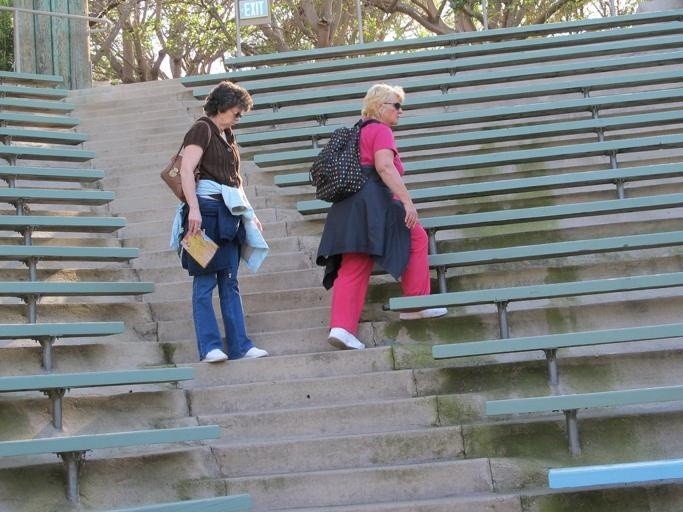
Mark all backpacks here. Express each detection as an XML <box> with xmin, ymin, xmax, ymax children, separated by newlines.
<box><xmin>310</xmin><ymin>118</ymin><xmax>381</xmax><ymax>203</ymax></box>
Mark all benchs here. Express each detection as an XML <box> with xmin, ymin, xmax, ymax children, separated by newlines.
<box><xmin>0</xmin><ymin>10</ymin><xmax>683</xmax><ymax>512</ymax></box>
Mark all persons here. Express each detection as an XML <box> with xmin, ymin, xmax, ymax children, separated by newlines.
<box><xmin>327</xmin><ymin>84</ymin><xmax>448</xmax><ymax>350</ymax></box>
<box><xmin>159</xmin><ymin>82</ymin><xmax>269</xmax><ymax>363</ymax></box>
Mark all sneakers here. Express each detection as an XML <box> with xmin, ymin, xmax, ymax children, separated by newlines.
<box><xmin>400</xmin><ymin>307</ymin><xmax>448</xmax><ymax>320</ymax></box>
<box><xmin>327</xmin><ymin>326</ymin><xmax>365</xmax><ymax>348</ymax></box>
<box><xmin>202</xmin><ymin>348</ymin><xmax>228</xmax><ymax>362</ymax></box>
<box><xmin>244</xmin><ymin>347</ymin><xmax>269</xmax><ymax>358</ymax></box>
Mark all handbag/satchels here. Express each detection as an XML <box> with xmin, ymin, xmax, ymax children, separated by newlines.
<box><xmin>161</xmin><ymin>155</ymin><xmax>200</xmax><ymax>202</ymax></box>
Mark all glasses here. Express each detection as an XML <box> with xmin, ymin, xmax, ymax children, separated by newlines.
<box><xmin>385</xmin><ymin>102</ymin><xmax>401</xmax><ymax>109</ymax></box>
<box><xmin>231</xmin><ymin>109</ymin><xmax>243</xmax><ymax>118</ymax></box>
